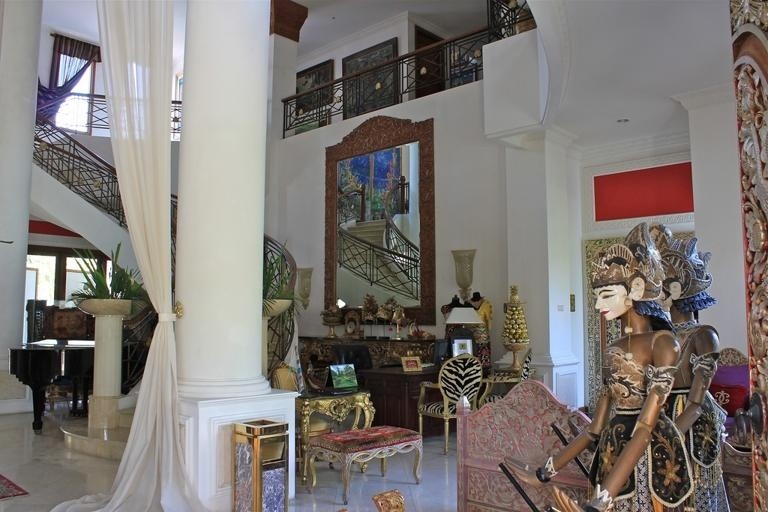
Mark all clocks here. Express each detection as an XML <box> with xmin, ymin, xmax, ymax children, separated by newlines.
<box><xmin>342</xmin><ymin>311</ymin><xmax>361</xmax><ymax>340</ymax></box>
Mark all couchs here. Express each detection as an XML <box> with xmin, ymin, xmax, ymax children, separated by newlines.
<box><xmin>453</xmin><ymin>378</ymin><xmax>762</xmax><ymax>512</ymax></box>
<box><xmin>707</xmin><ymin>348</ymin><xmax>749</xmax><ymax>436</ymax></box>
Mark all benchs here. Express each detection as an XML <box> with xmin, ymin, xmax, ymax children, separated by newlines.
<box><xmin>296</xmin><ymin>388</ymin><xmax>425</xmax><ymax>505</ymax></box>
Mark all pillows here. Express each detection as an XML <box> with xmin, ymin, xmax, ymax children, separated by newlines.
<box><xmin>708</xmin><ymin>385</ymin><xmax>747</xmax><ymax>416</ymax></box>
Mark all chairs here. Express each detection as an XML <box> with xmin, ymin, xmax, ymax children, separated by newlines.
<box><xmin>271</xmin><ymin>366</ymin><xmax>335</xmax><ymax>474</ymax></box>
<box><xmin>478</xmin><ymin>347</ymin><xmax>532</xmax><ymax>409</ymax></box>
<box><xmin>417</xmin><ymin>353</ymin><xmax>492</xmax><ymax>456</ymax></box>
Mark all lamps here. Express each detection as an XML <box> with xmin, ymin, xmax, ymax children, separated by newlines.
<box><xmin>446</xmin><ymin>307</ymin><xmax>484</xmax><ymax>329</ymax></box>
<box><xmin>451</xmin><ymin>249</ymin><xmax>476</xmax><ymax>301</ymax></box>
<box><xmin>296</xmin><ymin>267</ymin><xmax>314</xmax><ymax>309</ymax></box>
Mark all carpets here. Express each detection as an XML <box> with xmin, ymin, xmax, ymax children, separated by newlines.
<box><xmin>0</xmin><ymin>473</ymin><xmax>30</xmax><ymax>501</ymax></box>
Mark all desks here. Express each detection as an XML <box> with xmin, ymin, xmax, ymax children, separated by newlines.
<box><xmin>358</xmin><ymin>364</ymin><xmax>451</xmax><ymax>438</ymax></box>
<box><xmin>297</xmin><ymin>335</ymin><xmax>443</xmax><ymax>392</ymax></box>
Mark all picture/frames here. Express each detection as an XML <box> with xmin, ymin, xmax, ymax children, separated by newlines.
<box><xmin>324</xmin><ymin>115</ymin><xmax>436</xmax><ymax>326</ymax></box>
<box><xmin>342</xmin><ymin>36</ymin><xmax>399</xmax><ymax>120</ymax></box>
<box><xmin>295</xmin><ymin>59</ymin><xmax>334</xmax><ymax>116</ymax></box>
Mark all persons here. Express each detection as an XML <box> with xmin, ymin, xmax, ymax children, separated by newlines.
<box><xmin>503</xmin><ymin>221</ymin><xmax>691</xmax><ymax>512</ymax></box>
<box><xmin>569</xmin><ymin>224</ymin><xmax>731</xmax><ymax>512</ymax></box>
